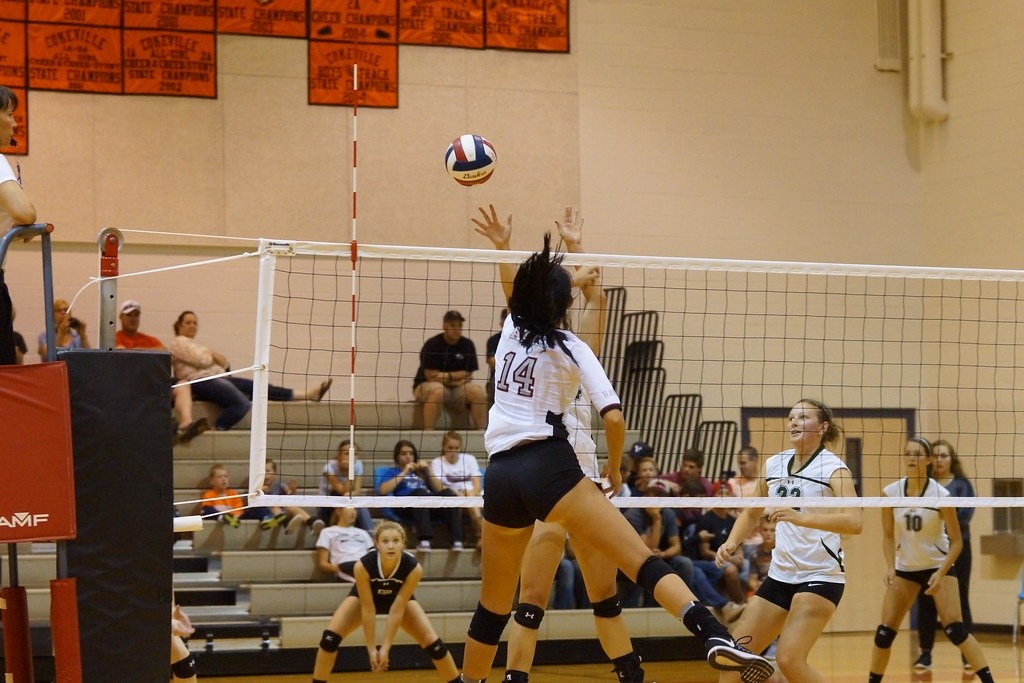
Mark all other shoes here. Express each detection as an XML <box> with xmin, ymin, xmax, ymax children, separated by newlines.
<box><xmin>286</xmin><ymin>514</ymin><xmax>302</xmax><ymax>535</ymax></box>
<box><xmin>311</xmin><ymin>519</ymin><xmax>325</xmax><ymax>536</ymax></box>
<box><xmin>222</xmin><ymin>513</ymin><xmax>240</xmax><ymax>528</ymax></box>
<box><xmin>762</xmin><ymin>640</ymin><xmax>778</xmax><ymax>660</ymax></box>
<box><xmin>452</xmin><ymin>541</ymin><xmax>463</xmax><ymax>551</ymax></box>
<box><xmin>962</xmin><ymin>655</ymin><xmax>972</xmax><ymax>668</ymax></box>
<box><xmin>914</xmin><ymin>652</ymin><xmax>931</xmax><ymax>668</ymax></box>
<box><xmin>416</xmin><ymin>542</ymin><xmax>431</xmax><ymax>552</ymax></box>
<box><xmin>261</xmin><ymin>513</ymin><xmax>287</xmax><ymax>530</ymax></box>
<box><xmin>722</xmin><ymin>601</ymin><xmax>748</xmax><ymax>624</ymax></box>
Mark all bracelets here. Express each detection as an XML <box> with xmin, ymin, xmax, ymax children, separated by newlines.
<box><xmin>449</xmin><ymin>373</ymin><xmax>452</xmax><ymax>380</ymax></box>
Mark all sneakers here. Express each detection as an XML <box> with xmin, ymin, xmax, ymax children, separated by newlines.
<box><xmin>705</xmin><ymin>635</ymin><xmax>774</xmax><ymax>683</ymax></box>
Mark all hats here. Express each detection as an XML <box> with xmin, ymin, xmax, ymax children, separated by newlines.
<box><xmin>622</xmin><ymin>441</ymin><xmax>654</xmax><ymax>458</ymax></box>
<box><xmin>121</xmin><ymin>300</ymin><xmax>142</xmax><ymax>314</ymax></box>
<box><xmin>445</xmin><ymin>310</ymin><xmax>465</xmax><ymax>323</ymax></box>
<box><xmin>713</xmin><ymin>482</ymin><xmax>732</xmax><ymax>497</ymax></box>
<box><xmin>647</xmin><ymin>480</ymin><xmax>670</xmax><ymax>494</ymax></box>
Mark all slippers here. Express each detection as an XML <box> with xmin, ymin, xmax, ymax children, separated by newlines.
<box><xmin>318</xmin><ymin>379</ymin><xmax>331</xmax><ymax>401</ymax></box>
<box><xmin>180</xmin><ymin>418</ymin><xmax>209</xmax><ymax>443</ymax></box>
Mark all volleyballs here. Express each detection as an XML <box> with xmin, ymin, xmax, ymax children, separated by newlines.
<box><xmin>444</xmin><ymin>133</ymin><xmax>497</xmax><ymax>187</ymax></box>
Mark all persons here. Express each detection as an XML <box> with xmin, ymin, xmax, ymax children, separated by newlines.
<box><xmin>485</xmin><ymin>309</ymin><xmax>508</xmax><ymax>408</ymax></box>
<box><xmin>171</xmin><ymin>604</ymin><xmax>199</xmax><ymax>683</ymax></box>
<box><xmin>470</xmin><ymin>204</ymin><xmax>645</xmax><ymax>683</ymax></box>
<box><xmin>0</xmin><ymin>86</ymin><xmax>36</xmax><ymax>365</ymax></box>
<box><xmin>115</xmin><ymin>302</ymin><xmax>209</xmax><ymax>442</ymax></box>
<box><xmin>412</xmin><ymin>310</ymin><xmax>488</xmax><ymax>430</ymax></box>
<box><xmin>199</xmin><ymin>464</ymin><xmax>287</xmax><ymax>531</ymax></box>
<box><xmin>913</xmin><ymin>441</ymin><xmax>976</xmax><ymax>667</ymax></box>
<box><xmin>313</xmin><ymin>521</ymin><xmax>462</xmax><ymax>683</ymax></box>
<box><xmin>37</xmin><ymin>298</ymin><xmax>90</xmax><ymax>362</ymax></box>
<box><xmin>868</xmin><ymin>438</ymin><xmax>995</xmax><ymax>683</ymax></box>
<box><xmin>170</xmin><ymin>312</ymin><xmax>333</xmax><ymax>430</ymax></box>
<box><xmin>459</xmin><ymin>235</ymin><xmax>775</xmax><ymax>683</ymax></box>
<box><xmin>262</xmin><ymin>458</ymin><xmax>326</xmax><ymax>536</ymax></box>
<box><xmin>316</xmin><ymin>430</ymin><xmax>484</xmax><ymax>585</ymax></box>
<box><xmin>553</xmin><ymin>442</ymin><xmax>775</xmax><ymax>622</ymax></box>
<box><xmin>715</xmin><ymin>399</ymin><xmax>863</xmax><ymax>683</ymax></box>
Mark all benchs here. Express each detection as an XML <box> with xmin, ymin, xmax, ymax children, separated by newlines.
<box><xmin>0</xmin><ymin>396</ymin><xmax>739</xmax><ymax>652</ymax></box>
